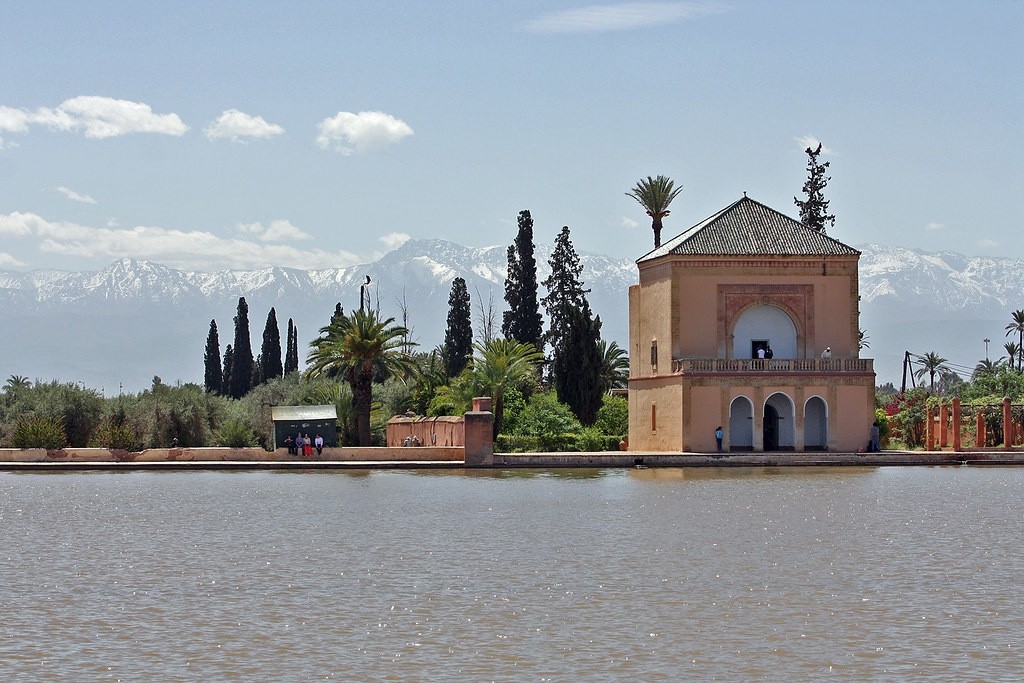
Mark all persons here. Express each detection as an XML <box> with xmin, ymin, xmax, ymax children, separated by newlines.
<box><xmin>757</xmin><ymin>345</ymin><xmax>773</xmax><ymax>359</ymax></box>
<box><xmin>170</xmin><ymin>438</ymin><xmax>178</xmax><ymax>448</ymax></box>
<box><xmin>404</xmin><ymin>436</ymin><xmax>419</xmax><ymax>447</ymax></box>
<box><xmin>715</xmin><ymin>426</ymin><xmax>723</xmax><ymax>451</ymax></box>
<box><xmin>871</xmin><ymin>422</ymin><xmax>881</xmax><ymax>453</ymax></box>
<box><xmin>284</xmin><ymin>433</ymin><xmax>323</xmax><ymax>457</ymax></box>
<box><xmin>619</xmin><ymin>435</ymin><xmax>628</xmax><ymax>451</ymax></box>
<box><xmin>821</xmin><ymin>347</ymin><xmax>832</xmax><ymax>359</ymax></box>
